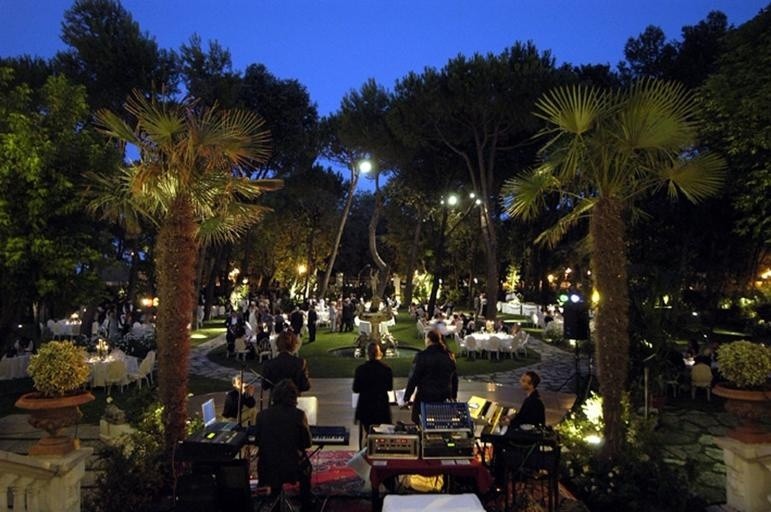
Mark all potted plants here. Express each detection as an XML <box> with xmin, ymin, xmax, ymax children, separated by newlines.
<box><xmin>713</xmin><ymin>340</ymin><xmax>771</xmax><ymax>443</ymax></box>
<box><xmin>10</xmin><ymin>340</ymin><xmax>95</xmax><ymax>458</ymax></box>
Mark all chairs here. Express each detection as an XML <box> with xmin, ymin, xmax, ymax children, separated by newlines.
<box><xmin>455</xmin><ymin>327</ymin><xmax>530</xmax><ymax>360</ymax></box>
<box><xmin>513</xmin><ymin>438</ymin><xmax>562</xmax><ymax>511</ymax></box>
<box><xmin>76</xmin><ymin>348</ymin><xmax>156</xmax><ymax>396</ymax></box>
<box><xmin>234</xmin><ymin>334</ymin><xmax>271</xmax><ymax>364</ymax></box>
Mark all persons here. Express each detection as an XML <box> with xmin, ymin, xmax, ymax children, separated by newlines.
<box><xmin>640</xmin><ymin>333</ymin><xmax>718</xmax><ymax>401</ymax></box>
<box><xmin>2</xmin><ymin>305</ymin><xmax>157</xmax><ymax>359</ymax></box>
<box><xmin>198</xmin><ymin>292</ymin><xmax>563</xmax><ymax>512</ymax></box>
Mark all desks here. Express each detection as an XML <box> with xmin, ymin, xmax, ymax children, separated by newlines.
<box><xmin>372</xmin><ymin>458</ymin><xmax>478</xmax><ymax>511</ymax></box>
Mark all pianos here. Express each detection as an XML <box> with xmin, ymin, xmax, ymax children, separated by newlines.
<box><xmin>241</xmin><ymin>425</ymin><xmax>349</xmax><ymax>445</ymax></box>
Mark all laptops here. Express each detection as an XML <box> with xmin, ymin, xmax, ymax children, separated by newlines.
<box><xmin>201</xmin><ymin>398</ymin><xmax>236</xmax><ymax>432</ymax></box>
